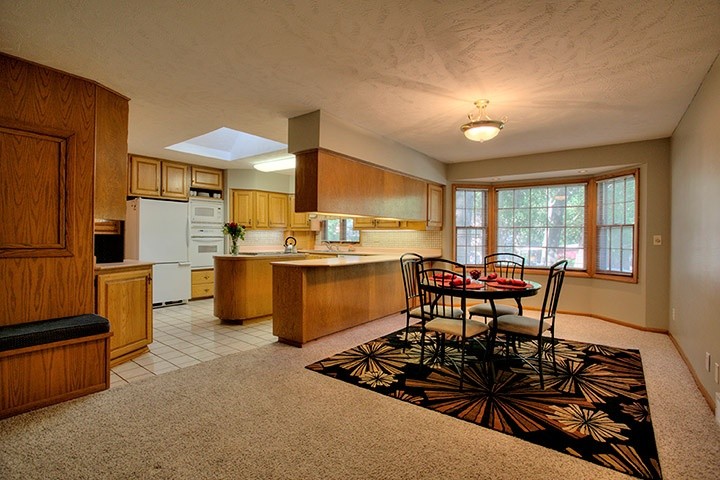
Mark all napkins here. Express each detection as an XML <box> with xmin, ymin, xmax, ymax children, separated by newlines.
<box><xmin>433</xmin><ymin>273</ymin><xmax>458</xmax><ymax>280</ymax></box>
<box><xmin>487</xmin><ymin>271</ymin><xmax>499</xmax><ymax>279</ymax></box>
<box><xmin>449</xmin><ymin>279</ymin><xmax>470</xmax><ymax>288</ymax></box>
<box><xmin>496</xmin><ymin>278</ymin><xmax>526</xmax><ymax>286</ymax></box>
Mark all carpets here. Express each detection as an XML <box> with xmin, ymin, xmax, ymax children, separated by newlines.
<box><xmin>303</xmin><ymin>319</ymin><xmax>664</xmax><ymax>480</ymax></box>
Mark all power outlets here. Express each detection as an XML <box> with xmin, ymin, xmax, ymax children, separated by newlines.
<box><xmin>704</xmin><ymin>352</ymin><xmax>711</xmax><ymax>372</ymax></box>
<box><xmin>715</xmin><ymin>361</ymin><xmax>719</xmax><ymax>384</ymax></box>
<box><xmin>672</xmin><ymin>308</ymin><xmax>675</xmax><ymax>321</ymax></box>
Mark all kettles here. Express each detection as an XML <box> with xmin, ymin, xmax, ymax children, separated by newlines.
<box><xmin>283</xmin><ymin>236</ymin><xmax>297</xmax><ymax>254</ymax></box>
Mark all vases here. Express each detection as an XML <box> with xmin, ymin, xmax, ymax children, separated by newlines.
<box><xmin>231</xmin><ymin>240</ymin><xmax>239</xmax><ymax>254</ymax></box>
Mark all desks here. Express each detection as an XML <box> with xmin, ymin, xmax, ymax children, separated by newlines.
<box><xmin>417</xmin><ymin>276</ymin><xmax>540</xmax><ymax>386</ymax></box>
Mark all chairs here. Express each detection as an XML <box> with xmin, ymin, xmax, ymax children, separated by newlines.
<box><xmin>399</xmin><ymin>251</ymin><xmax>570</xmax><ymax>391</ymax></box>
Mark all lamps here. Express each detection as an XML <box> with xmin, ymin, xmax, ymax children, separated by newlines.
<box><xmin>253</xmin><ymin>154</ymin><xmax>297</xmax><ymax>173</ymax></box>
<box><xmin>459</xmin><ymin>98</ymin><xmax>510</xmax><ymax>143</ymax></box>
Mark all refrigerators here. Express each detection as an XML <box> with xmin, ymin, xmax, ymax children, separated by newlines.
<box><xmin>124</xmin><ymin>197</ymin><xmax>192</xmax><ymax>308</ymax></box>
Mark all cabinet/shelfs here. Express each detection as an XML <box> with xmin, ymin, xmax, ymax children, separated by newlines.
<box><xmin>291</xmin><ymin>194</ymin><xmax>311</xmax><ymax>228</ymax></box>
<box><xmin>354</xmin><ymin>218</ymin><xmax>401</xmax><ymax>231</ymax></box>
<box><xmin>190</xmin><ymin>167</ymin><xmax>226</xmax><ymax>200</ymax></box>
<box><xmin>254</xmin><ymin>191</ymin><xmax>269</xmax><ymax>229</ymax></box>
<box><xmin>235</xmin><ymin>191</ymin><xmax>254</xmax><ymax>227</ymax></box>
<box><xmin>129</xmin><ymin>157</ymin><xmax>191</xmax><ymax>200</ymax></box>
<box><xmin>270</xmin><ymin>193</ymin><xmax>288</xmax><ymax>227</ymax></box>
<box><xmin>192</xmin><ymin>269</ymin><xmax>217</xmax><ymax>299</ymax></box>
<box><xmin>100</xmin><ymin>269</ymin><xmax>155</xmax><ymax>359</ymax></box>
<box><xmin>426</xmin><ymin>182</ymin><xmax>443</xmax><ymax>230</ymax></box>
<box><xmin>399</xmin><ymin>221</ymin><xmax>425</xmax><ymax>229</ymax></box>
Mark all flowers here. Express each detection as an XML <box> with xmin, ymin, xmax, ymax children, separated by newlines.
<box><xmin>221</xmin><ymin>223</ymin><xmax>248</xmax><ymax>241</ymax></box>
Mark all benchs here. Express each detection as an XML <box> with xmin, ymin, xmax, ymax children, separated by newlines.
<box><xmin>1</xmin><ymin>315</ymin><xmax>115</xmax><ymax>422</ymax></box>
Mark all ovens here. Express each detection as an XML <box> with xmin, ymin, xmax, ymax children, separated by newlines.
<box><xmin>189</xmin><ymin>197</ymin><xmax>225</xmax><ymax>269</ymax></box>
